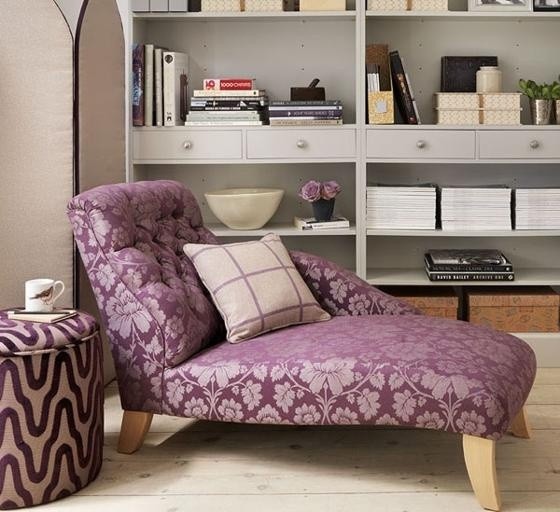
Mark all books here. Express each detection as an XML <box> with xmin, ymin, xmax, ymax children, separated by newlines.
<box><xmin>293</xmin><ymin>214</ymin><xmax>352</xmax><ymax>231</ymax></box>
<box><xmin>129</xmin><ymin>38</ymin><xmax>345</xmax><ymax>134</ymax></box>
<box><xmin>423</xmin><ymin>263</ymin><xmax>515</xmax><ymax>282</ymax></box>
<box><xmin>7</xmin><ymin>310</ymin><xmax>79</xmax><ymax>324</ymax></box>
<box><xmin>428</xmin><ymin>247</ymin><xmax>504</xmax><ymax>265</ymax></box>
<box><xmin>423</xmin><ymin>251</ymin><xmax>514</xmax><ymax>272</ymax></box>
<box><xmin>367</xmin><ymin>50</ymin><xmax>422</xmax><ymax>124</ymax></box>
<box><xmin>366</xmin><ymin>184</ymin><xmax>560</xmax><ymax>230</ymax></box>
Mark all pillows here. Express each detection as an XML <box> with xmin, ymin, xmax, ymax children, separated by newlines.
<box><xmin>182</xmin><ymin>232</ymin><xmax>331</xmax><ymax>344</ymax></box>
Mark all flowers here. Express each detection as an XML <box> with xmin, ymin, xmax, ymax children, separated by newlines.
<box><xmin>297</xmin><ymin>179</ymin><xmax>343</xmax><ymax>203</ymax></box>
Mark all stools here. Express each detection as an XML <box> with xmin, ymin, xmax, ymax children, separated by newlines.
<box><xmin>0</xmin><ymin>308</ymin><xmax>104</xmax><ymax>510</ymax></box>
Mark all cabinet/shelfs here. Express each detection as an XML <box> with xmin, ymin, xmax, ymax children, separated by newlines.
<box><xmin>365</xmin><ymin>10</ymin><xmax>560</xmax><ymax>287</ymax></box>
<box><xmin>133</xmin><ymin>11</ymin><xmax>356</xmax><ymax>238</ymax></box>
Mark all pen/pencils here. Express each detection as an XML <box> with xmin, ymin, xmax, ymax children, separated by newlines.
<box><xmin>15</xmin><ymin>311</ymin><xmax>71</xmax><ymax>314</ymax></box>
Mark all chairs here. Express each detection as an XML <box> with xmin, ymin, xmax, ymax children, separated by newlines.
<box><xmin>68</xmin><ymin>179</ymin><xmax>537</xmax><ymax>512</ymax></box>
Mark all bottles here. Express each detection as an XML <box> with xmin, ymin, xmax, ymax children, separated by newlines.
<box><xmin>475</xmin><ymin>65</ymin><xmax>502</xmax><ymax>95</ymax></box>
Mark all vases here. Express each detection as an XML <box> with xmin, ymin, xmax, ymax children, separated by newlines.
<box><xmin>312</xmin><ymin>197</ymin><xmax>335</xmax><ymax>220</ymax></box>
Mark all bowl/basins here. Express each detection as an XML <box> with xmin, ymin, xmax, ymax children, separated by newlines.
<box><xmin>204</xmin><ymin>187</ymin><xmax>285</xmax><ymax>230</ymax></box>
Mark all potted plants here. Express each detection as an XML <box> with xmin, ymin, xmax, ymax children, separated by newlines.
<box><xmin>519</xmin><ymin>74</ymin><xmax>560</xmax><ymax>125</ymax></box>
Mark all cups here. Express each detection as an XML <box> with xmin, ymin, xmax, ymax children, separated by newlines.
<box><xmin>25</xmin><ymin>279</ymin><xmax>65</xmax><ymax>313</ymax></box>
